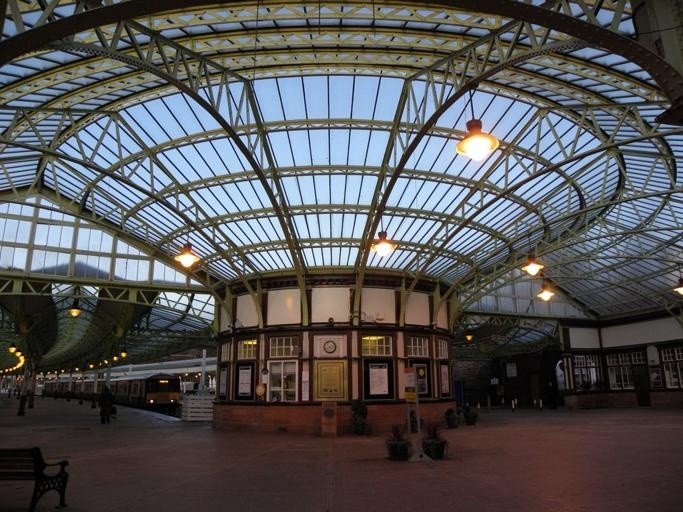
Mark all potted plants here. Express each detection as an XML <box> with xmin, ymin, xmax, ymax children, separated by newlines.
<box><xmin>353</xmin><ymin>396</ymin><xmax>479</xmax><ymax>463</ymax></box>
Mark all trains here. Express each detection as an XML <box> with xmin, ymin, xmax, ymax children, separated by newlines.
<box><xmin>41</xmin><ymin>372</ymin><xmax>181</xmax><ymax>416</ymax></box>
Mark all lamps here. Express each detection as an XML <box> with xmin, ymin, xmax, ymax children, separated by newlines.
<box><xmin>673</xmin><ymin>265</ymin><xmax>683</xmax><ymax>296</ymax></box>
<box><xmin>67</xmin><ymin>298</ymin><xmax>82</xmax><ymax>318</ymax></box>
<box><xmin>174</xmin><ymin>225</ymin><xmax>200</xmax><ymax>268</ymax></box>
<box><xmin>367</xmin><ymin>212</ymin><xmax>400</xmax><ymax>257</ymax></box>
<box><xmin>466</xmin><ymin>332</ymin><xmax>474</xmax><ymax>341</ymax></box>
<box><xmin>456</xmin><ymin>84</ymin><xmax>498</xmax><ymax>161</ymax></box>
<box><xmin>518</xmin><ymin>230</ymin><xmax>555</xmax><ymax>303</ymax></box>
<box><xmin>9</xmin><ymin>344</ymin><xmax>22</xmax><ymax>357</ymax></box>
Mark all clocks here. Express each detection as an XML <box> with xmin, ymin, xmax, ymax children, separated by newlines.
<box><xmin>324</xmin><ymin>340</ymin><xmax>336</xmax><ymax>354</ymax></box>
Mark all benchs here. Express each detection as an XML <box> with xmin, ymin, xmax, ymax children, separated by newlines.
<box><xmin>1</xmin><ymin>446</ymin><xmax>70</xmax><ymax>512</ymax></box>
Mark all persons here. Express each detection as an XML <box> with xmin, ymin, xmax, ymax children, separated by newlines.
<box><xmin>97</xmin><ymin>384</ymin><xmax>114</xmax><ymax>425</ymax></box>
